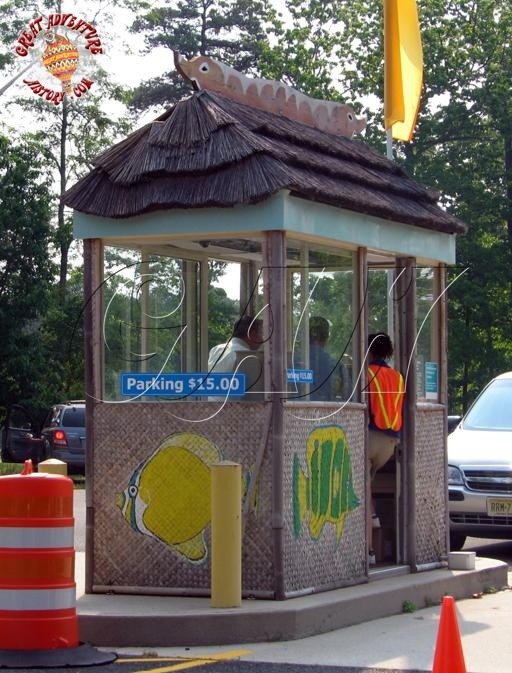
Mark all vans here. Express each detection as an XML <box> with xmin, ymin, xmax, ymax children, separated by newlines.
<box><xmin>1</xmin><ymin>399</ymin><xmax>86</xmax><ymax>475</ymax></box>
<box><xmin>446</xmin><ymin>371</ymin><xmax>512</xmax><ymax>551</ymax></box>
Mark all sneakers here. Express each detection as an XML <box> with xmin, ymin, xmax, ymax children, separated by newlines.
<box><xmin>369</xmin><ymin>550</ymin><xmax>376</xmax><ymax>564</ymax></box>
<box><xmin>371</xmin><ymin>514</ymin><xmax>380</xmax><ymax>528</ymax></box>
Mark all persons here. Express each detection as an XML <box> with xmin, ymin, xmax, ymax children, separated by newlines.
<box><xmin>208</xmin><ymin>315</ymin><xmax>264</xmax><ymax>399</ymax></box>
<box><xmin>20</xmin><ymin>423</ymin><xmax>31</xmax><ymax>435</ymax></box>
<box><xmin>286</xmin><ymin>317</ymin><xmax>352</xmax><ymax>402</ymax></box>
<box><xmin>367</xmin><ymin>332</ymin><xmax>406</xmax><ymax>564</ymax></box>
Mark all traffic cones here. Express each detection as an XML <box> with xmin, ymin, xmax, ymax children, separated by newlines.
<box><xmin>432</xmin><ymin>596</ymin><xmax>467</xmax><ymax>673</ymax></box>
